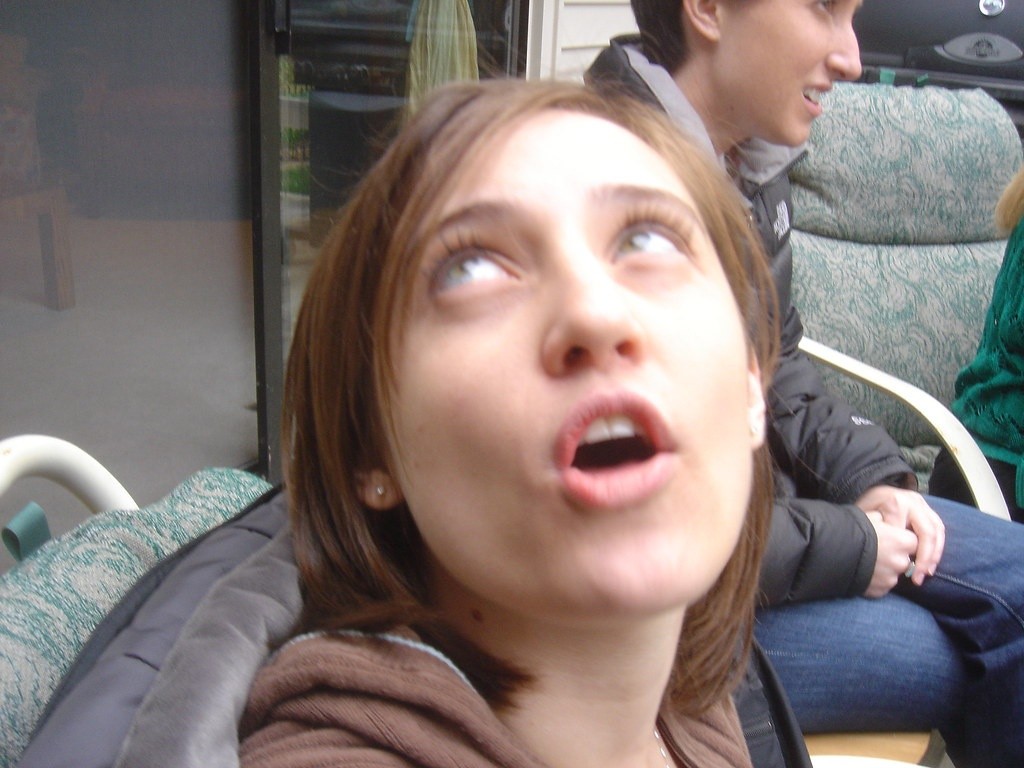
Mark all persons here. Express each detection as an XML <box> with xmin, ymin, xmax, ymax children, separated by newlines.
<box><xmin>929</xmin><ymin>168</ymin><xmax>1024</xmax><ymax>526</ymax></box>
<box><xmin>242</xmin><ymin>74</ymin><xmax>783</xmax><ymax>768</ymax></box>
<box><xmin>579</xmin><ymin>0</ymin><xmax>1024</xmax><ymax>768</ymax></box>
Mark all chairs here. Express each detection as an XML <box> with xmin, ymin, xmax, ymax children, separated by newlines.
<box><xmin>0</xmin><ymin>83</ymin><xmax>1024</xmax><ymax>768</ymax></box>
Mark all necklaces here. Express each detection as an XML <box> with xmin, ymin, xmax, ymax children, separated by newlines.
<box><xmin>653</xmin><ymin>731</ymin><xmax>670</xmax><ymax>768</ymax></box>
